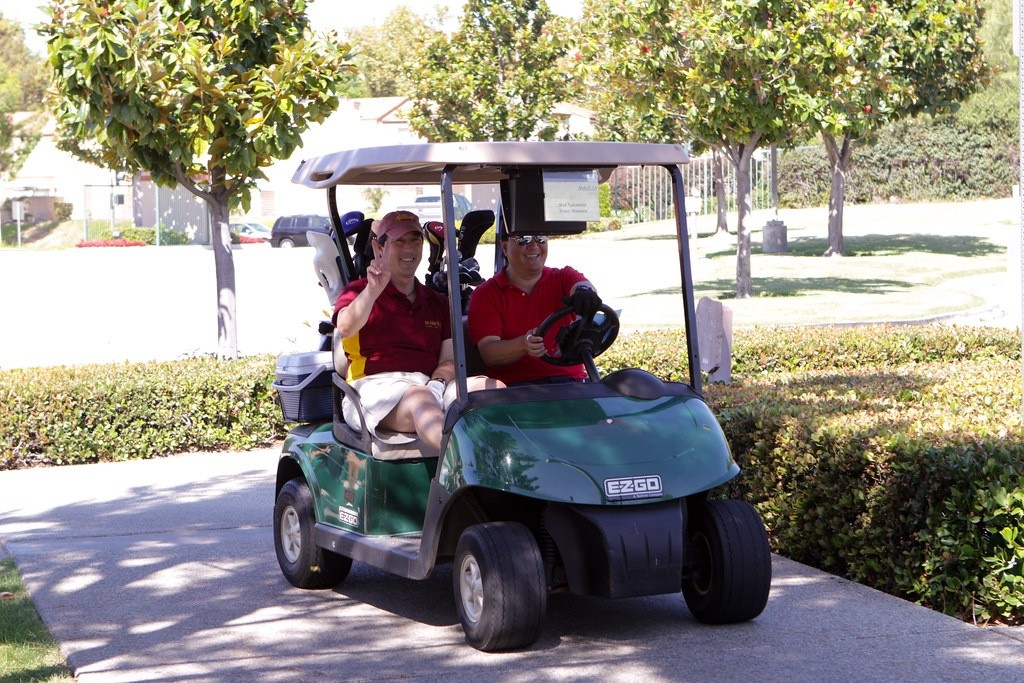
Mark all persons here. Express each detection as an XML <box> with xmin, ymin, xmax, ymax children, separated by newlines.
<box><xmin>332</xmin><ymin>211</ymin><xmax>508</xmax><ymax>452</ymax></box>
<box><xmin>468</xmin><ymin>214</ymin><xmax>597</xmax><ymax>387</ymax></box>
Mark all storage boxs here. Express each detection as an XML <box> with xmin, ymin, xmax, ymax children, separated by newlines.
<box><xmin>274</xmin><ymin>351</ymin><xmax>335</xmax><ymax>423</ymax></box>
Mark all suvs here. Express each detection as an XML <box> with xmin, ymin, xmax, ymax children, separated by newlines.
<box><xmin>269</xmin><ymin>214</ymin><xmax>356</xmax><ymax>249</ymax></box>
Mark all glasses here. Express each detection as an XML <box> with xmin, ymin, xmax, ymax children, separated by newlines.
<box><xmin>508</xmin><ymin>236</ymin><xmax>548</xmax><ymax>246</ymax></box>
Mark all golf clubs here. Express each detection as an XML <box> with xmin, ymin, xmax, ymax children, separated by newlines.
<box><xmin>423</xmin><ymin>208</ymin><xmax>496</xmax><ymax>314</ymax></box>
<box><xmin>329</xmin><ymin>210</ymin><xmax>366</xmax><ymax>288</ymax></box>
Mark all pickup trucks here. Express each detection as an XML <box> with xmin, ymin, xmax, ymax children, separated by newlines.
<box><xmin>395</xmin><ymin>194</ymin><xmax>473</xmax><ymax>223</ymax></box>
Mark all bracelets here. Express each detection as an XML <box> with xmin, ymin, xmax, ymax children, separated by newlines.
<box><xmin>574</xmin><ymin>285</ymin><xmax>590</xmax><ymax>292</ymax></box>
<box><xmin>432</xmin><ymin>376</ymin><xmax>447</xmax><ymax>390</ymax></box>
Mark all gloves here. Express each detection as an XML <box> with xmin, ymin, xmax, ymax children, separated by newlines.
<box><xmin>426</xmin><ymin>381</ymin><xmax>445</xmax><ymax>410</ymax></box>
<box><xmin>561</xmin><ymin>286</ymin><xmax>601</xmax><ymax>318</ymax></box>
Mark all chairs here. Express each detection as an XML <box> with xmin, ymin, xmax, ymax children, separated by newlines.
<box><xmin>330</xmin><ymin>328</ymin><xmax>438</xmax><ymax>460</ymax></box>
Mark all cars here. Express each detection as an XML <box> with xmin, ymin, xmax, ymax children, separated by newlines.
<box><xmin>229</xmin><ymin>222</ymin><xmax>273</xmax><ymax>243</ymax></box>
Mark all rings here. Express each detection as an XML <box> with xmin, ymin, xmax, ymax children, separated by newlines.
<box><xmin>371</xmin><ymin>260</ymin><xmax>375</xmax><ymax>265</ymax></box>
<box><xmin>526</xmin><ymin>334</ymin><xmax>532</xmax><ymax>341</ymax></box>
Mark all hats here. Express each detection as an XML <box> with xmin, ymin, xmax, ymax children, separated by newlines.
<box><xmin>371</xmin><ymin>211</ymin><xmax>424</xmax><ymax>240</ymax></box>
<box><xmin>500</xmin><ymin>220</ymin><xmax>506</xmax><ymax>238</ymax></box>
